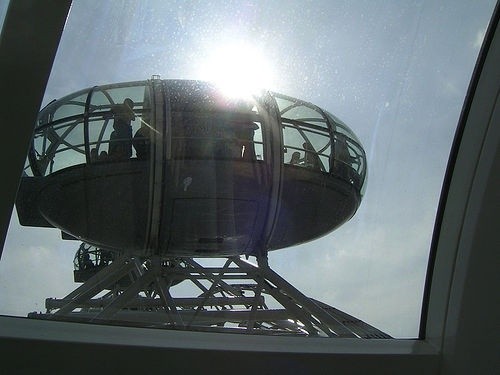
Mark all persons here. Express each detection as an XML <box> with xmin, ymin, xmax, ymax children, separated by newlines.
<box><xmin>90</xmin><ymin>148</ymin><xmax>101</xmax><ymax>162</ymax></box>
<box><xmin>99</xmin><ymin>151</ymin><xmax>107</xmax><ymax>162</ymax></box>
<box><xmin>290</xmin><ymin>152</ymin><xmax>301</xmax><ymax>165</ymax></box>
<box><xmin>328</xmin><ymin>133</ymin><xmax>352</xmax><ymax>170</ymax></box>
<box><xmin>303</xmin><ymin>142</ymin><xmax>316</xmax><ymax>169</ymax></box>
<box><xmin>103</xmin><ymin>98</ymin><xmax>136</xmax><ymax>162</ymax></box>
<box><xmin>237</xmin><ymin>99</ymin><xmax>257</xmax><ymax>160</ymax></box>
